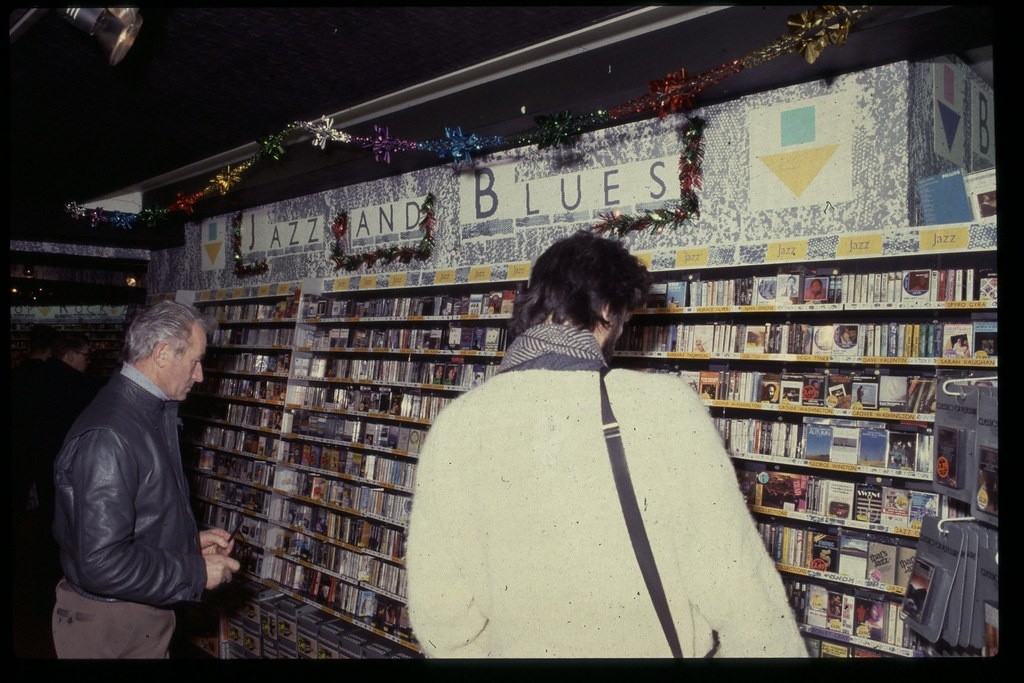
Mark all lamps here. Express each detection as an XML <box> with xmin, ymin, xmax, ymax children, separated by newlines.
<box><xmin>10</xmin><ymin>8</ymin><xmax>143</xmax><ymax>66</ymax></box>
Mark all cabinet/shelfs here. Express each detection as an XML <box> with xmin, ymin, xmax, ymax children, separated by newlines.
<box><xmin>11</xmin><ymin>220</ymin><xmax>998</xmax><ymax>659</ymax></box>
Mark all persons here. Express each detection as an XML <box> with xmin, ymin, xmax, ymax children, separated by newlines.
<box><xmin>404</xmin><ymin>226</ymin><xmax>816</xmax><ymax>659</ymax></box>
<box><xmin>7</xmin><ymin>321</ymin><xmax>93</xmax><ymax>578</ymax></box>
<box><xmin>433</xmin><ymin>364</ymin><xmax>459</xmax><ymax>385</ymax></box>
<box><xmin>48</xmin><ymin>299</ymin><xmax>243</xmax><ymax>660</ymax></box>
<box><xmin>761</xmin><ymin>382</ymin><xmax>778</xmax><ymax>404</ymax></box>
<box><xmin>826</xmin><ymin>593</ymin><xmax>842</xmax><ymax>619</ymax></box>
<box><xmin>856</xmin><ymin>384</ymin><xmax>865</xmax><ymax>408</ymax></box>
<box><xmin>861</xmin><ymin>605</ymin><xmax>884</xmax><ymax>642</ymax></box>
<box><xmin>667</xmin><ymin>296</ymin><xmax>680</xmax><ymax>308</ymax></box>
<box><xmin>952</xmin><ymin>336</ymin><xmax>968</xmax><ymax>357</ymax></box>
<box><xmin>776</xmin><ymin>275</ymin><xmax>826</xmax><ymax>301</ymax></box>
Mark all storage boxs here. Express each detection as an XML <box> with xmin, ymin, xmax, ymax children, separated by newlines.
<box><xmin>297</xmin><ymin>609</ymin><xmax>337</xmax><ymax>659</ymax></box>
<box><xmin>227</xmin><ymin>573</ymin><xmax>252</xmax><ymax>659</ymax></box>
<box><xmin>338</xmin><ymin>628</ymin><xmax>384</xmax><ymax>659</ymax></box>
<box><xmin>278</xmin><ymin>597</ymin><xmax>307</xmax><ymax>659</ymax></box>
<box><xmin>362</xmin><ymin>638</ymin><xmax>407</xmax><ymax>659</ymax></box>
<box><xmin>317</xmin><ymin>618</ymin><xmax>360</xmax><ymax>659</ymax></box>
<box><xmin>243</xmin><ymin>580</ymin><xmax>285</xmax><ymax>659</ymax></box>
<box><xmin>261</xmin><ymin>595</ymin><xmax>289</xmax><ymax>659</ymax></box>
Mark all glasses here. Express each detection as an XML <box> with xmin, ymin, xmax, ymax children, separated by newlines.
<box><xmin>74</xmin><ymin>349</ymin><xmax>90</xmax><ymax>358</ymax></box>
